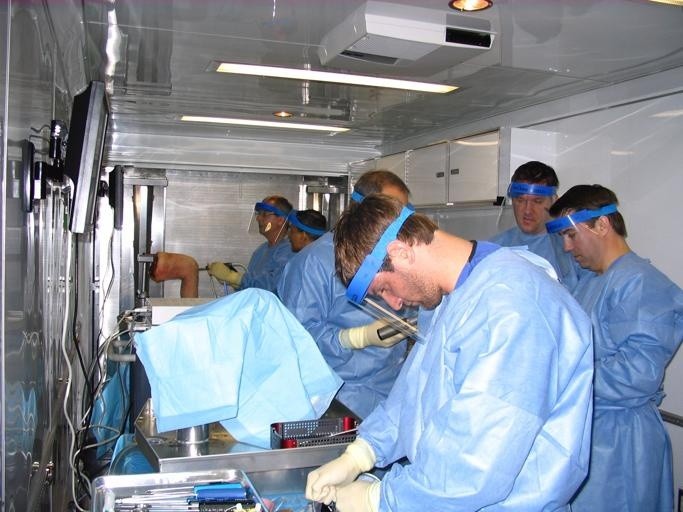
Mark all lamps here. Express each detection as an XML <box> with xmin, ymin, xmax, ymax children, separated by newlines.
<box><xmin>448</xmin><ymin>0</ymin><xmax>493</xmax><ymax>12</ymax></box>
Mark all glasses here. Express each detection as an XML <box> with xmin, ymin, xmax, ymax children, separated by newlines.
<box><xmin>254</xmin><ymin>211</ymin><xmax>276</xmax><ymax>217</ymax></box>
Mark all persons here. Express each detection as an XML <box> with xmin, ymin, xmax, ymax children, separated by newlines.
<box><xmin>484</xmin><ymin>160</ymin><xmax>594</xmax><ymax>295</ymax></box>
<box><xmin>278</xmin><ymin>170</ymin><xmax>419</xmax><ymax>418</ymax></box>
<box><xmin>207</xmin><ymin>196</ymin><xmax>298</xmax><ymax>296</ymax></box>
<box><xmin>547</xmin><ymin>183</ymin><xmax>682</xmax><ymax>511</ymax></box>
<box><xmin>304</xmin><ymin>193</ymin><xmax>595</xmax><ymax>512</ymax></box>
<box><xmin>290</xmin><ymin>209</ymin><xmax>326</xmax><ymax>252</ymax></box>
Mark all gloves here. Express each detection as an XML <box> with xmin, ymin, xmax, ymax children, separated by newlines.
<box><xmin>209</xmin><ymin>261</ymin><xmax>242</xmax><ymax>289</ymax></box>
<box><xmin>335</xmin><ymin>480</ymin><xmax>379</xmax><ymax>512</ymax></box>
<box><xmin>306</xmin><ymin>437</ymin><xmax>377</xmax><ymax>505</ymax></box>
<box><xmin>339</xmin><ymin>319</ymin><xmax>415</xmax><ymax>349</ymax></box>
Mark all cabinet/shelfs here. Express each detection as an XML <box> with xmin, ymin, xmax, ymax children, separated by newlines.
<box><xmin>405</xmin><ymin>126</ymin><xmax>557</xmax><ymax>210</ymax></box>
<box><xmin>348</xmin><ymin>151</ymin><xmax>405</xmax><ymax>204</ymax></box>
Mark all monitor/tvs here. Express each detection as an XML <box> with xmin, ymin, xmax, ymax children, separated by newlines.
<box><xmin>110</xmin><ymin>165</ymin><xmax>123</xmax><ymax>229</ymax></box>
<box><xmin>65</xmin><ymin>81</ymin><xmax>110</xmax><ymax>237</ymax></box>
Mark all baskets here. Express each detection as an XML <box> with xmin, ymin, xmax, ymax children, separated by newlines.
<box><xmin>269</xmin><ymin>416</ymin><xmax>360</xmax><ymax>449</ymax></box>
